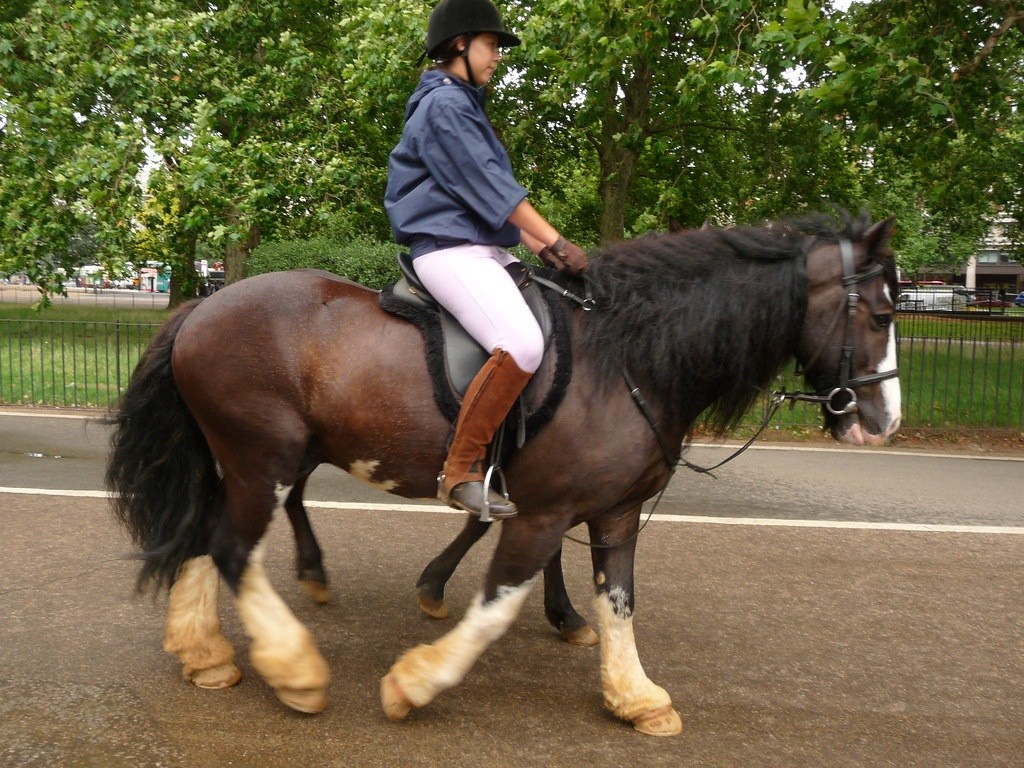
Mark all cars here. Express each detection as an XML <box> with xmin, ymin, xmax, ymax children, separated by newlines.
<box><xmin>975</xmin><ymin>296</ymin><xmax>1012</xmax><ymax>308</ymax></box>
<box><xmin>74</xmin><ymin>263</ymin><xmax>139</xmax><ymax>289</ymax></box>
<box><xmin>965</xmin><ymin>296</ymin><xmax>977</xmax><ymax>307</ymax></box>
<box><xmin>1014</xmin><ymin>291</ymin><xmax>1024</xmax><ymax>308</ymax></box>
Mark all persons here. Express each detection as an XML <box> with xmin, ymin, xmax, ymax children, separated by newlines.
<box><xmin>385</xmin><ymin>0</ymin><xmax>588</xmax><ymax>518</ymax></box>
<box><xmin>213</xmin><ymin>260</ymin><xmax>224</xmax><ymax>271</ymax></box>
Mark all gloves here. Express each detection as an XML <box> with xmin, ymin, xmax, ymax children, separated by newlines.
<box><xmin>550</xmin><ymin>235</ymin><xmax>590</xmax><ymax>276</ymax></box>
<box><xmin>536</xmin><ymin>245</ymin><xmax>566</xmax><ymax>271</ymax></box>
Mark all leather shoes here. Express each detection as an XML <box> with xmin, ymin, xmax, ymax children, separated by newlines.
<box><xmin>437</xmin><ymin>477</ymin><xmax>518</xmax><ymax>518</ymax></box>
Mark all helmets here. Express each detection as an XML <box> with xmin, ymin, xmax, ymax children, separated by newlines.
<box><xmin>427</xmin><ymin>0</ymin><xmax>522</xmax><ymax>59</ymax></box>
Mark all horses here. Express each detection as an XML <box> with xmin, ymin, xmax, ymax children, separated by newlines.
<box><xmin>109</xmin><ymin>220</ymin><xmax>905</xmax><ymax>736</ymax></box>
